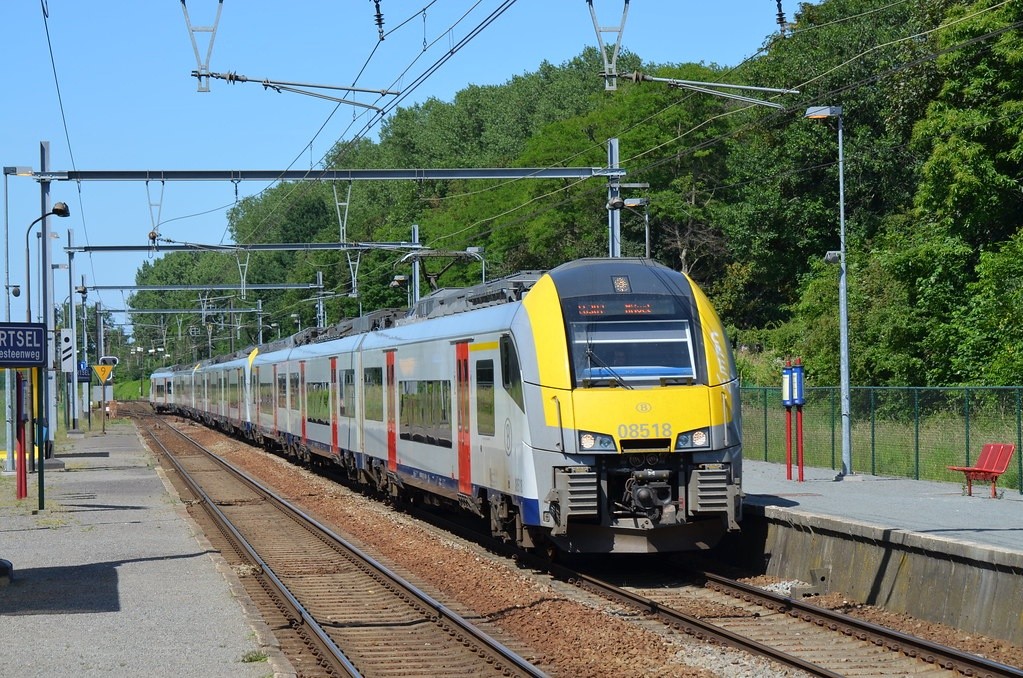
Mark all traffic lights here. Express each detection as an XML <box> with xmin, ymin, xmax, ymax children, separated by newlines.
<box><xmin>99</xmin><ymin>356</ymin><xmax>119</xmax><ymax>380</ymax></box>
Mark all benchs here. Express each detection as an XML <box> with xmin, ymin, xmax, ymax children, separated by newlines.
<box><xmin>948</xmin><ymin>444</ymin><xmax>1015</xmax><ymax>498</ymax></box>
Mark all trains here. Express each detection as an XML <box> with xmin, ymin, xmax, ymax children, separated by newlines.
<box><xmin>148</xmin><ymin>256</ymin><xmax>746</xmax><ymax>569</ymax></box>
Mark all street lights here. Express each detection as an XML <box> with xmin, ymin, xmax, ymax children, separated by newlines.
<box><xmin>62</xmin><ymin>284</ymin><xmax>88</xmax><ymax>428</ymax></box>
<box><xmin>290</xmin><ymin>313</ymin><xmax>301</xmax><ymax>332</ymax></box>
<box><xmin>130</xmin><ymin>346</ymin><xmax>143</xmax><ymax>397</ymax></box>
<box><xmin>388</xmin><ymin>274</ymin><xmax>411</xmax><ymax>308</ymax></box>
<box><xmin>606</xmin><ymin>196</ymin><xmax>652</xmax><ymax>261</ymax></box>
<box><xmin>29</xmin><ymin>202</ymin><xmax>71</xmax><ymax>474</ymax></box>
<box><xmin>270</xmin><ymin>322</ymin><xmax>280</xmax><ymax>339</ymax></box>
<box><xmin>805</xmin><ymin>102</ymin><xmax>854</xmax><ymax>475</ymax></box>
<box><xmin>465</xmin><ymin>247</ymin><xmax>486</xmax><ymax>284</ymax></box>
